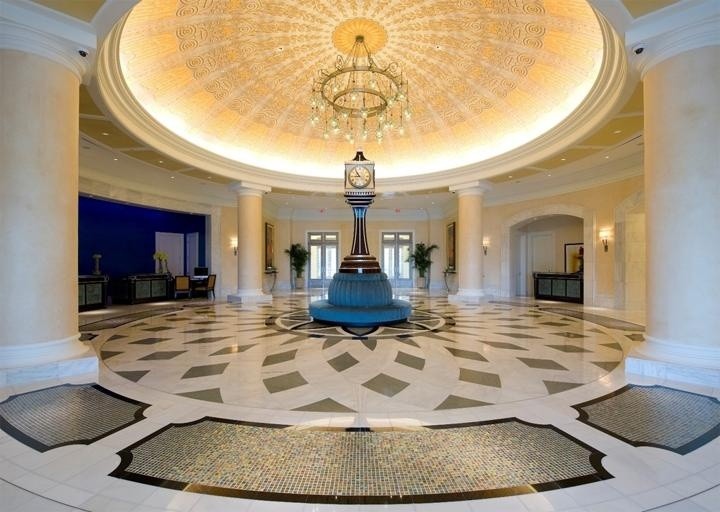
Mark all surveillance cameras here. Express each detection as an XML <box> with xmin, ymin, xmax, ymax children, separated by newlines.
<box><xmin>633</xmin><ymin>46</ymin><xmax>645</xmax><ymax>56</ymax></box>
<box><xmin>78</xmin><ymin>49</ymin><xmax>89</xmax><ymax>57</ymax></box>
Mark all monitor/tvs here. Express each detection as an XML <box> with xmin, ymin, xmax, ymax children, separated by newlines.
<box><xmin>194</xmin><ymin>267</ymin><xmax>208</xmax><ymax>276</ymax></box>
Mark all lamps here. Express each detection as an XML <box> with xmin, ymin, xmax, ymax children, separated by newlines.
<box><xmin>310</xmin><ymin>34</ymin><xmax>416</xmax><ymax>151</ymax></box>
<box><xmin>597</xmin><ymin>226</ymin><xmax>612</xmax><ymax>253</ymax></box>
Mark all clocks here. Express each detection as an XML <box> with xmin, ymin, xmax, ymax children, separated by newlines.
<box><xmin>343</xmin><ymin>161</ymin><xmax>374</xmax><ymax>196</ymax></box>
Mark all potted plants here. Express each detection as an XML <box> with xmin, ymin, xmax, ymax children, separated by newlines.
<box><xmin>404</xmin><ymin>241</ymin><xmax>441</xmax><ymax>288</ymax></box>
<box><xmin>286</xmin><ymin>242</ymin><xmax>312</xmax><ymax>293</ymax></box>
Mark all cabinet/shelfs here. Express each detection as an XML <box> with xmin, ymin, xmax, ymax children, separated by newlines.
<box><xmin>77</xmin><ymin>274</ymin><xmax>109</xmax><ymax>312</ymax></box>
<box><xmin>534</xmin><ymin>270</ymin><xmax>585</xmax><ymax>302</ymax></box>
<box><xmin>122</xmin><ymin>271</ymin><xmax>173</xmax><ymax>303</ymax></box>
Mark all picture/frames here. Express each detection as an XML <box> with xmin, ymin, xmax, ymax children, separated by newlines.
<box><xmin>264</xmin><ymin>222</ymin><xmax>276</xmax><ymax>270</ymax></box>
<box><xmin>444</xmin><ymin>221</ymin><xmax>457</xmax><ymax>271</ymax></box>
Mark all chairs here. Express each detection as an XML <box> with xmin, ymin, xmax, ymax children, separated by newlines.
<box><xmin>170</xmin><ymin>268</ymin><xmax>219</xmax><ymax>302</ymax></box>
